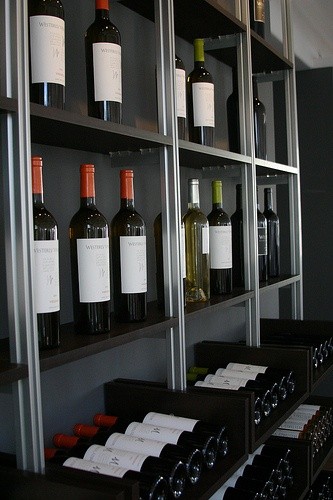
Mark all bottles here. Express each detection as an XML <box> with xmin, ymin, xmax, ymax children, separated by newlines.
<box><xmin>107</xmin><ymin>168</ymin><xmax>148</xmax><ymax>325</ymax></box>
<box><xmin>184</xmin><ymin>37</ymin><xmax>215</xmax><ymax>148</ymax></box>
<box><xmin>156</xmin><ymin>54</ymin><xmax>187</xmax><ymax>142</ymax></box>
<box><xmin>42</xmin><ymin>324</ymin><xmax>333</xmax><ymax>500</ymax></box>
<box><xmin>30</xmin><ymin>155</ymin><xmax>62</xmax><ymax>351</ymax></box>
<box><xmin>207</xmin><ymin>181</ymin><xmax>234</xmax><ymax>297</ymax></box>
<box><xmin>248</xmin><ymin>0</ymin><xmax>267</xmax><ymax>40</ymax></box>
<box><xmin>227</xmin><ymin>69</ymin><xmax>268</xmax><ymax>162</ymax></box>
<box><xmin>181</xmin><ymin>178</ymin><xmax>212</xmax><ymax>305</ymax></box>
<box><xmin>152</xmin><ymin>210</ymin><xmax>167</xmax><ymax>316</ymax></box>
<box><xmin>231</xmin><ymin>184</ymin><xmax>280</xmax><ymax>291</ymax></box>
<box><xmin>84</xmin><ymin>0</ymin><xmax>128</xmax><ymax>126</ymax></box>
<box><xmin>26</xmin><ymin>1</ymin><xmax>69</xmax><ymax>110</ymax></box>
<box><xmin>67</xmin><ymin>163</ymin><xmax>113</xmax><ymax>338</ymax></box>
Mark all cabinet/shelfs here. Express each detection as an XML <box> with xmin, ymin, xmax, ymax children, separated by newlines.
<box><xmin>0</xmin><ymin>0</ymin><xmax>333</xmax><ymax>500</ymax></box>
